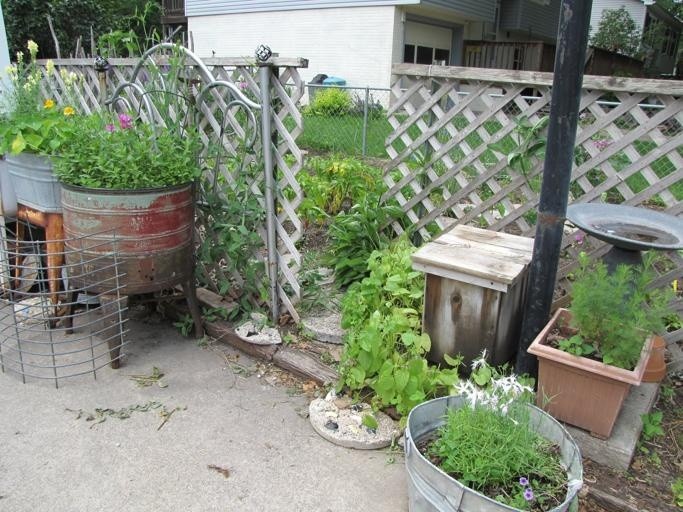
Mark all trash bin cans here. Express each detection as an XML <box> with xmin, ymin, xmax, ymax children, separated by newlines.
<box><xmin>308</xmin><ymin>74</ymin><xmax>346</xmax><ymax>106</ymax></box>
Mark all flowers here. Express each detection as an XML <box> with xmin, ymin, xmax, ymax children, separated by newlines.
<box><xmin>431</xmin><ymin>349</ymin><xmax>570</xmax><ymax>512</ymax></box>
<box><xmin>0</xmin><ymin>39</ymin><xmax>198</xmax><ymax>189</ymax></box>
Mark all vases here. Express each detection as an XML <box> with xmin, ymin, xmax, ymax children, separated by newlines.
<box><xmin>5</xmin><ymin>153</ymin><xmax>65</xmax><ymax>218</ymax></box>
<box><xmin>59</xmin><ymin>183</ymin><xmax>198</xmax><ymax>297</ymax></box>
<box><xmin>1</xmin><ymin>160</ymin><xmax>18</xmax><ymax>225</ymax></box>
<box><xmin>404</xmin><ymin>392</ymin><xmax>584</xmax><ymax>512</ymax></box>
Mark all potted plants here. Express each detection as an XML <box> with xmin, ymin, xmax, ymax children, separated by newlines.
<box><xmin>527</xmin><ymin>247</ymin><xmax>683</xmax><ymax>441</ymax></box>
